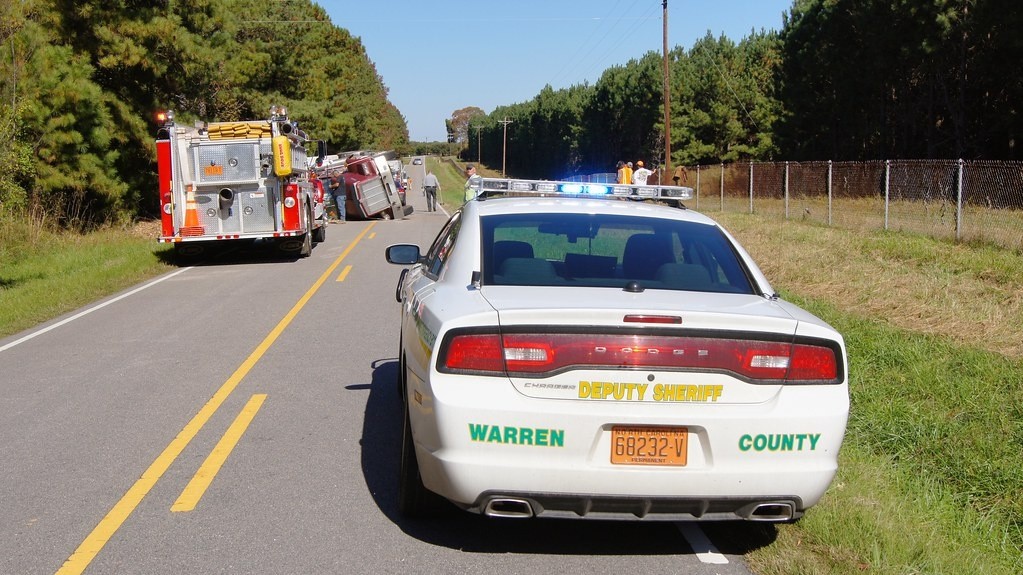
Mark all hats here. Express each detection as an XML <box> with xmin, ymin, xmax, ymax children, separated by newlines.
<box><xmin>466</xmin><ymin>164</ymin><xmax>475</xmax><ymax>169</ymax></box>
<box><xmin>636</xmin><ymin>161</ymin><xmax>643</xmax><ymax>166</ymax></box>
<box><xmin>616</xmin><ymin>161</ymin><xmax>624</xmax><ymax>167</ymax></box>
<box><xmin>627</xmin><ymin>162</ymin><xmax>633</xmax><ymax>168</ymax></box>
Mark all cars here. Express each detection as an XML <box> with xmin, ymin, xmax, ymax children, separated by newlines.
<box><xmin>413</xmin><ymin>159</ymin><xmax>422</xmax><ymax>165</ymax></box>
<box><xmin>395</xmin><ymin>181</ymin><xmax>406</xmax><ymax>206</ymax></box>
<box><xmin>385</xmin><ymin>175</ymin><xmax>850</xmax><ymax>522</ymax></box>
<box><xmin>402</xmin><ymin>174</ymin><xmax>412</xmax><ymax>190</ymax></box>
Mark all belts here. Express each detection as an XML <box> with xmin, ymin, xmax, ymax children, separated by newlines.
<box><xmin>426</xmin><ymin>186</ymin><xmax>436</xmax><ymax>188</ymax></box>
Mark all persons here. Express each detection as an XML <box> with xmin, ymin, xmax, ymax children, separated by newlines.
<box><xmin>615</xmin><ymin>161</ymin><xmax>656</xmax><ymax>203</ymax></box>
<box><xmin>672</xmin><ymin>165</ymin><xmax>687</xmax><ymax>186</ymax></box>
<box><xmin>329</xmin><ymin>170</ymin><xmax>346</xmax><ymax>220</ymax></box>
<box><xmin>463</xmin><ymin>164</ymin><xmax>482</xmax><ymax>204</ymax></box>
<box><xmin>424</xmin><ymin>170</ymin><xmax>441</xmax><ymax>212</ymax></box>
<box><xmin>407</xmin><ymin>176</ymin><xmax>412</xmax><ymax>190</ymax></box>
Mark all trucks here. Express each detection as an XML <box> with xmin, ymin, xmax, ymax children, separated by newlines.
<box><xmin>387</xmin><ymin>160</ymin><xmax>404</xmax><ymax>186</ymax></box>
<box><xmin>307</xmin><ymin>150</ymin><xmax>411</xmax><ymax>221</ymax></box>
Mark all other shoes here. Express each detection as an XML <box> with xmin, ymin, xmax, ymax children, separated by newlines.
<box><xmin>433</xmin><ymin>207</ymin><xmax>436</xmax><ymax>211</ymax></box>
<box><xmin>337</xmin><ymin>221</ymin><xmax>346</xmax><ymax>224</ymax></box>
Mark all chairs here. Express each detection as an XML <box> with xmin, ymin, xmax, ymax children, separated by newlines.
<box><xmin>494</xmin><ymin>241</ymin><xmax>535</xmax><ymax>276</ymax></box>
<box><xmin>498</xmin><ymin>258</ymin><xmax>555</xmax><ymax>279</ymax></box>
<box><xmin>656</xmin><ymin>263</ymin><xmax>712</xmax><ymax>286</ymax></box>
<box><xmin>622</xmin><ymin>234</ymin><xmax>671</xmax><ymax>280</ymax></box>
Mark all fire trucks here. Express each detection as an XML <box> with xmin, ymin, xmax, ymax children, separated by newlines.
<box><xmin>157</xmin><ymin>106</ymin><xmax>326</xmax><ymax>257</ymax></box>
